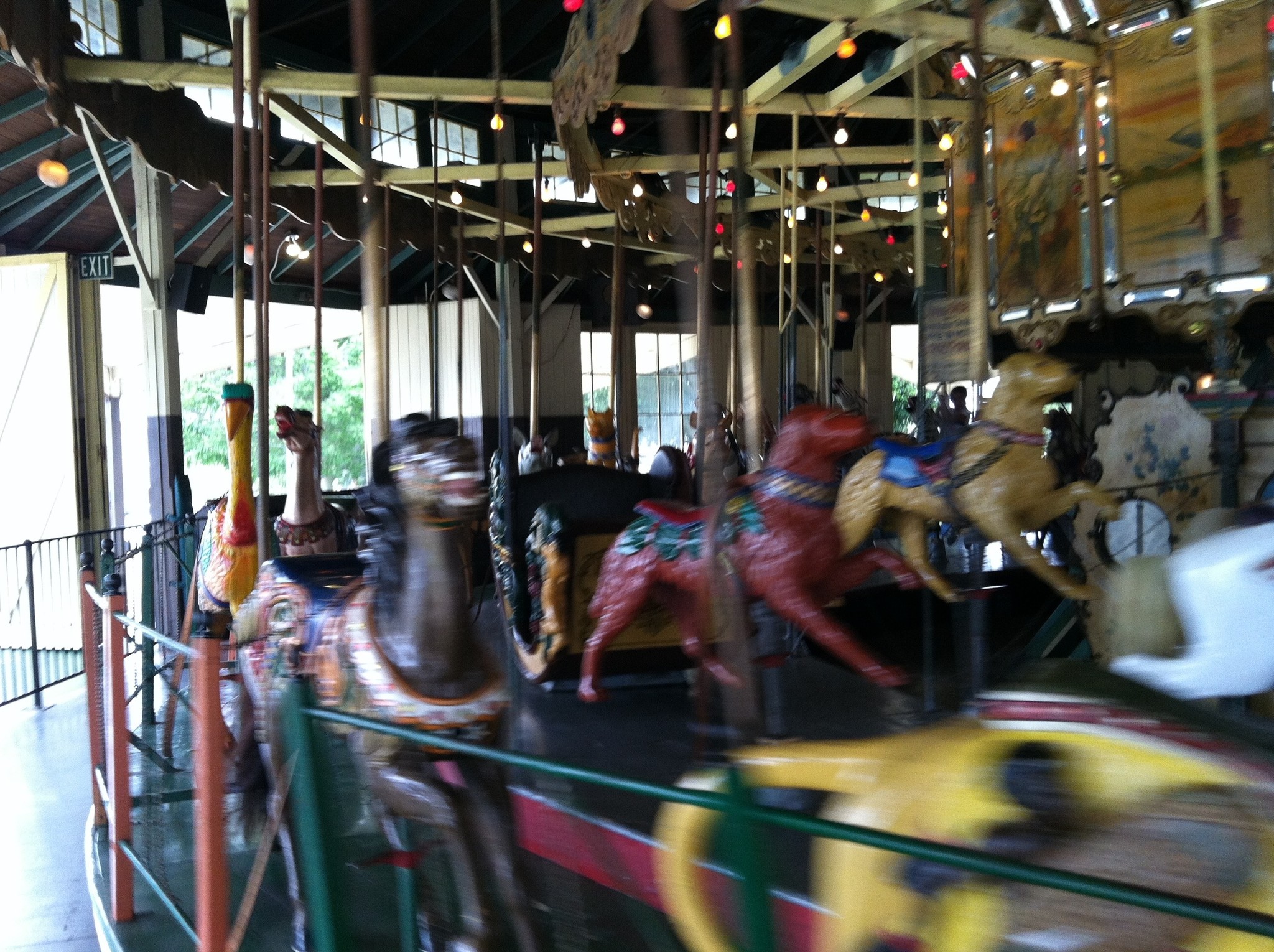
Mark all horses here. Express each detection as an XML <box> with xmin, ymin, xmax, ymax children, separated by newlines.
<box><xmin>236</xmin><ymin>404</ymin><xmax>549</xmax><ymax>952</ymax></box>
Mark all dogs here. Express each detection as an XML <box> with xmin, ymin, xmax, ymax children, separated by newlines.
<box><xmin>576</xmin><ymin>350</ymin><xmax>1124</xmax><ymax>705</ymax></box>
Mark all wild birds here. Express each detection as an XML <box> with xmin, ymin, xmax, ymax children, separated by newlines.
<box><xmin>157</xmin><ymin>380</ymin><xmax>275</xmax><ymax>748</ymax></box>
